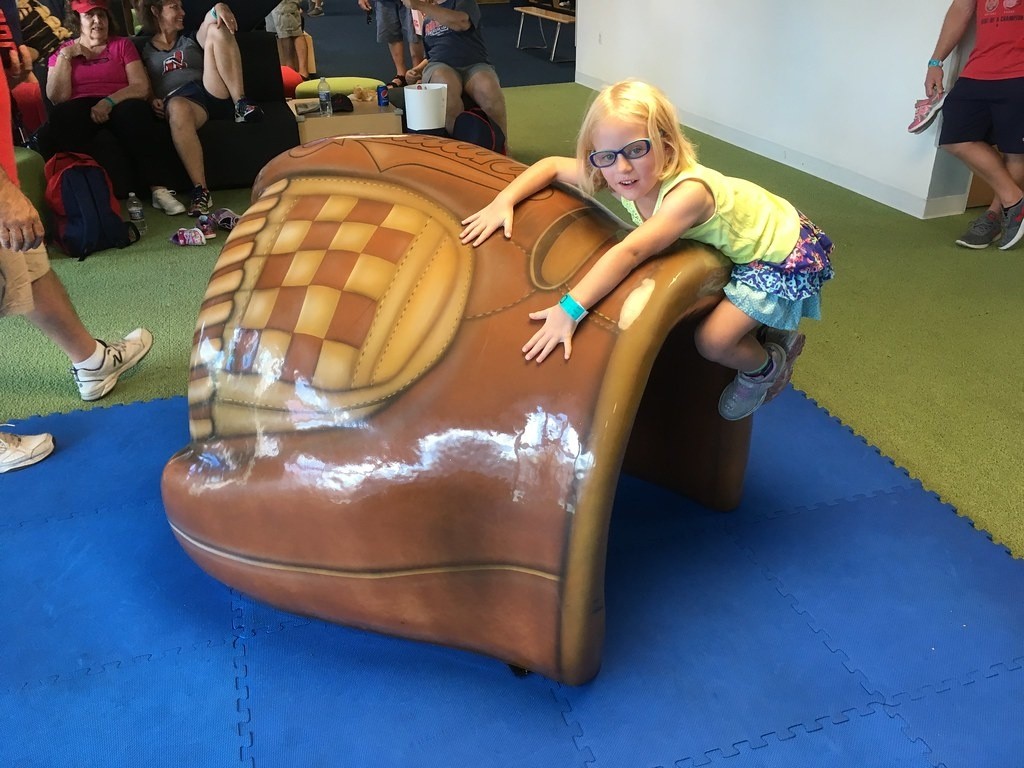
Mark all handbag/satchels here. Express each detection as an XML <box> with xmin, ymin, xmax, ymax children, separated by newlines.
<box><xmin>453</xmin><ymin>109</ymin><xmax>505</xmax><ymax>153</ymax></box>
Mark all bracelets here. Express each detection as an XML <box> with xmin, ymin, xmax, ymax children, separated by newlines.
<box><xmin>106</xmin><ymin>94</ymin><xmax>115</xmax><ymax>105</ymax></box>
<box><xmin>211</xmin><ymin>7</ymin><xmax>217</xmax><ymax>19</ymax></box>
<box><xmin>557</xmin><ymin>291</ymin><xmax>589</xmax><ymax>323</ymax></box>
<box><xmin>928</xmin><ymin>59</ymin><xmax>944</xmax><ymax>68</ymax></box>
<box><xmin>59</xmin><ymin>51</ymin><xmax>70</xmax><ymax>61</ymax></box>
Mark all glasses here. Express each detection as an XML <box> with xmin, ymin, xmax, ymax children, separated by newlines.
<box><xmin>82</xmin><ymin>42</ymin><xmax>109</xmax><ymax>65</ymax></box>
<box><xmin>367</xmin><ymin>7</ymin><xmax>373</xmax><ymax>25</ymax></box>
<box><xmin>589</xmin><ymin>130</ymin><xmax>664</xmax><ymax>168</ymax></box>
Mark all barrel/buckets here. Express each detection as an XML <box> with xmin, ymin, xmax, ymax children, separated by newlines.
<box><xmin>403</xmin><ymin>83</ymin><xmax>448</xmax><ymax>131</ymax></box>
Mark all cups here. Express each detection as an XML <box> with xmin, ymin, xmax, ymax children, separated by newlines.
<box><xmin>162</xmin><ymin>133</ymin><xmax>752</xmax><ymax>687</ymax></box>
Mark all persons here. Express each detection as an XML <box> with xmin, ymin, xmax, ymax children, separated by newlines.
<box><xmin>45</xmin><ymin>0</ymin><xmax>188</xmax><ymax>216</ymax></box>
<box><xmin>0</xmin><ymin>0</ymin><xmax>325</xmax><ymax>135</ymax></box>
<box><xmin>924</xmin><ymin>0</ymin><xmax>1024</xmax><ymax>251</ymax></box>
<box><xmin>459</xmin><ymin>77</ymin><xmax>834</xmax><ymax>422</ymax></box>
<box><xmin>130</xmin><ymin>0</ymin><xmax>265</xmax><ymax>217</ymax></box>
<box><xmin>0</xmin><ymin>59</ymin><xmax>154</xmax><ymax>474</ymax></box>
<box><xmin>357</xmin><ymin>0</ymin><xmax>507</xmax><ymax>155</ymax></box>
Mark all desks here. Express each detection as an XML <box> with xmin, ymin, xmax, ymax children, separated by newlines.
<box><xmin>286</xmin><ymin>97</ymin><xmax>403</xmax><ymax>146</ymax></box>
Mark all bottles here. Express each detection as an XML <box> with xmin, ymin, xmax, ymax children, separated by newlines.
<box><xmin>126</xmin><ymin>192</ymin><xmax>147</xmax><ymax>236</ymax></box>
<box><xmin>317</xmin><ymin>77</ymin><xmax>333</xmax><ymax>117</ymax></box>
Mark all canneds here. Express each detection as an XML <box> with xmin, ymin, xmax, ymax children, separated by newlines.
<box><xmin>377</xmin><ymin>85</ymin><xmax>389</xmax><ymax>106</ymax></box>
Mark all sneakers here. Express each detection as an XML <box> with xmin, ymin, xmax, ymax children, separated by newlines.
<box><xmin>908</xmin><ymin>88</ymin><xmax>948</xmax><ymax>135</ymax></box>
<box><xmin>718</xmin><ymin>325</ymin><xmax>806</xmax><ymax>421</ymax></box>
<box><xmin>187</xmin><ymin>184</ymin><xmax>213</xmax><ymax>217</ymax></box>
<box><xmin>955</xmin><ymin>210</ymin><xmax>1002</xmax><ymax>249</ymax></box>
<box><xmin>70</xmin><ymin>327</ymin><xmax>153</xmax><ymax>401</ymax></box>
<box><xmin>234</xmin><ymin>95</ymin><xmax>265</xmax><ymax>123</ymax></box>
<box><xmin>171</xmin><ymin>227</ymin><xmax>206</xmax><ymax>246</ymax></box>
<box><xmin>996</xmin><ymin>196</ymin><xmax>1024</xmax><ymax>251</ymax></box>
<box><xmin>194</xmin><ymin>208</ymin><xmax>242</xmax><ymax>239</ymax></box>
<box><xmin>0</xmin><ymin>424</ymin><xmax>56</xmax><ymax>474</ymax></box>
<box><xmin>152</xmin><ymin>189</ymin><xmax>186</xmax><ymax>216</ymax></box>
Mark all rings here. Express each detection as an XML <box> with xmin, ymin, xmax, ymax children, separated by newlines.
<box><xmin>229</xmin><ymin>19</ymin><xmax>233</xmax><ymax>22</ymax></box>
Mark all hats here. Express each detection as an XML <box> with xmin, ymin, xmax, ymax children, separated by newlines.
<box><xmin>71</xmin><ymin>0</ymin><xmax>107</xmax><ymax>13</ymax></box>
<box><xmin>331</xmin><ymin>93</ymin><xmax>353</xmax><ymax>112</ymax></box>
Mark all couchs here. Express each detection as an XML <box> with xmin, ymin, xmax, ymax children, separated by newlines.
<box><xmin>35</xmin><ymin>31</ymin><xmax>301</xmax><ymax>199</ymax></box>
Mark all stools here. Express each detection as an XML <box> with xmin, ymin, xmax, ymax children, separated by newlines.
<box><xmin>295</xmin><ymin>77</ymin><xmax>384</xmax><ymax>101</ymax></box>
<box><xmin>280</xmin><ymin>65</ymin><xmax>302</xmax><ymax>99</ymax></box>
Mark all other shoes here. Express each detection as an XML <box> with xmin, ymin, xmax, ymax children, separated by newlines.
<box><xmin>307</xmin><ymin>2</ymin><xmax>324</xmax><ymax>17</ymax></box>
<box><xmin>300</xmin><ymin>74</ymin><xmax>311</xmax><ymax>81</ymax></box>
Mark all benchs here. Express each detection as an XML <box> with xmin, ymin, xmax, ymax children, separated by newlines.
<box><xmin>513</xmin><ymin>6</ymin><xmax>575</xmax><ymax>62</ymax></box>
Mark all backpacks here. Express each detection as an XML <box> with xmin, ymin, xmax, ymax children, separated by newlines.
<box><xmin>44</xmin><ymin>151</ymin><xmax>140</xmax><ymax>261</ymax></box>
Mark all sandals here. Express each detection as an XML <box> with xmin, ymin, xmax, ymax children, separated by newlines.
<box><xmin>387</xmin><ymin>75</ymin><xmax>408</xmax><ymax>89</ymax></box>
